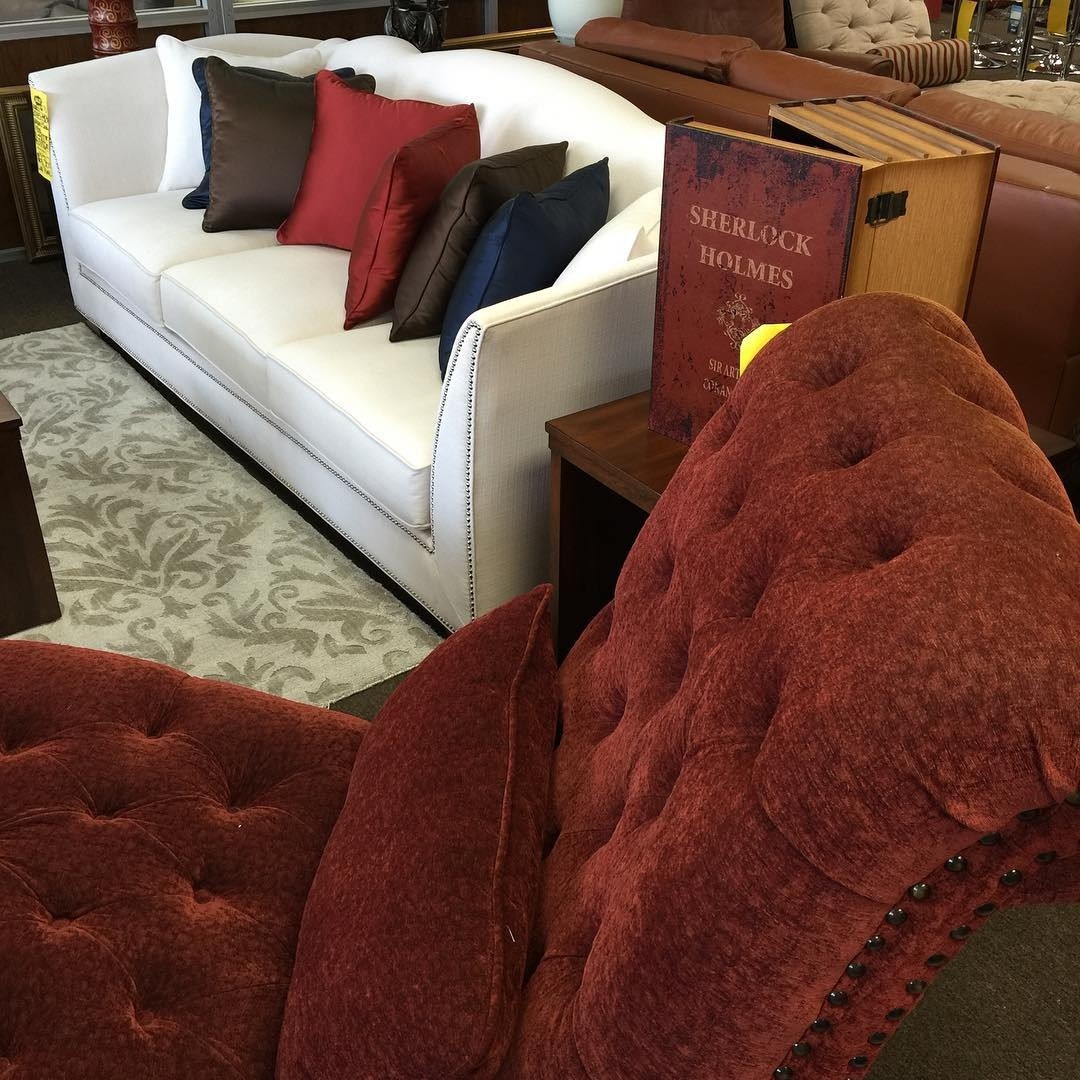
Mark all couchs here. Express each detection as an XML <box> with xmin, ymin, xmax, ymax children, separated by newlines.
<box><xmin>520</xmin><ymin>0</ymin><xmax>1080</xmax><ymax>436</ymax></box>
<box><xmin>28</xmin><ymin>30</ymin><xmax>666</xmax><ymax>641</ymax></box>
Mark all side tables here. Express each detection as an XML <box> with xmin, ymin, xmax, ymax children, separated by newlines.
<box><xmin>545</xmin><ymin>391</ymin><xmax>694</xmax><ymax>658</ymax></box>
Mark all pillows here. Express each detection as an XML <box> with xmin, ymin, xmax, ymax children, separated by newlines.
<box><xmin>273</xmin><ymin>582</ymin><xmax>552</xmax><ymax>1080</ymax></box>
<box><xmin>153</xmin><ymin>35</ymin><xmax>610</xmax><ymax>383</ymax></box>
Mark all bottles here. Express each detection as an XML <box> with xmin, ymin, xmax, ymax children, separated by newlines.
<box><xmin>1007</xmin><ymin>0</ymin><xmax>1023</xmax><ymax>33</ymax></box>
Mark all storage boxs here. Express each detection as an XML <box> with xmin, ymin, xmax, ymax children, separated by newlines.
<box><xmin>648</xmin><ymin>93</ymin><xmax>1001</xmax><ymax>448</ymax></box>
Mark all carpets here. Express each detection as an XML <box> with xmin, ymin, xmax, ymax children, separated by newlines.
<box><xmin>0</xmin><ymin>321</ymin><xmax>444</xmax><ymax>711</ymax></box>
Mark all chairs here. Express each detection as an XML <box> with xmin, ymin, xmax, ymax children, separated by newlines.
<box><xmin>0</xmin><ymin>293</ymin><xmax>1080</xmax><ymax>1080</ymax></box>
